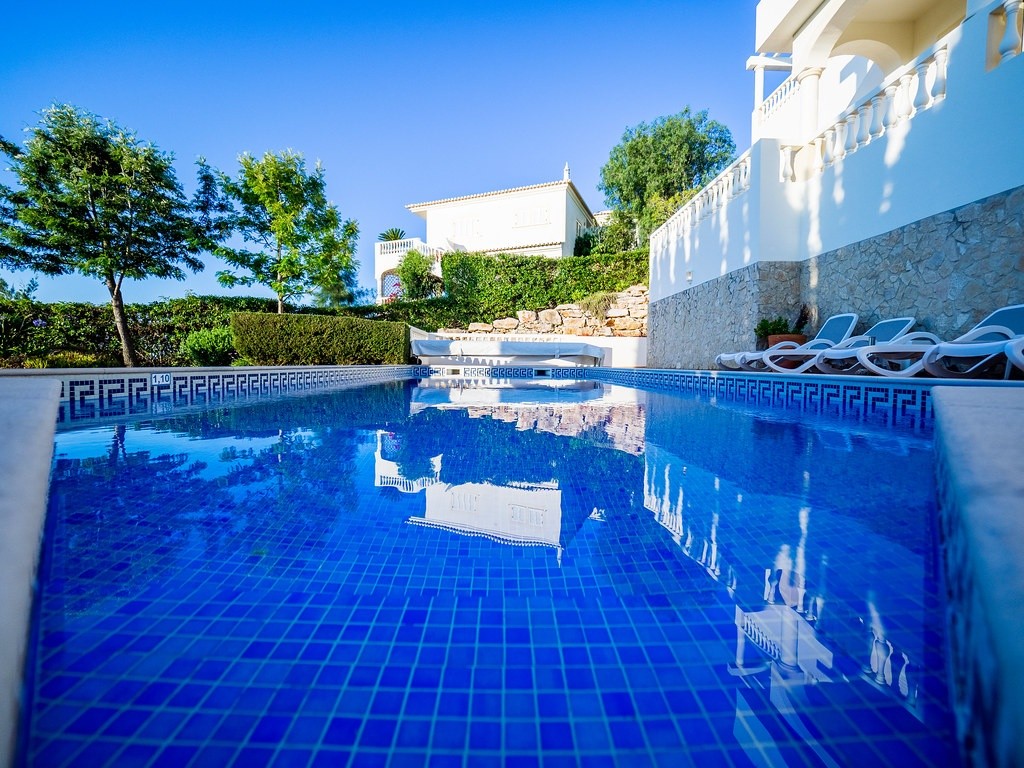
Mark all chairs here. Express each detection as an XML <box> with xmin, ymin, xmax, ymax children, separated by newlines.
<box><xmin>857</xmin><ymin>304</ymin><xmax>1024</xmax><ymax>377</ymax></box>
<box><xmin>715</xmin><ymin>313</ymin><xmax>859</xmax><ymax>372</ymax></box>
<box><xmin>762</xmin><ymin>317</ymin><xmax>917</xmax><ymax>374</ymax></box>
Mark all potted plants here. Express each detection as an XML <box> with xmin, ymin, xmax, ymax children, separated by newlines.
<box><xmin>754</xmin><ymin>303</ymin><xmax>813</xmax><ymax>372</ymax></box>
